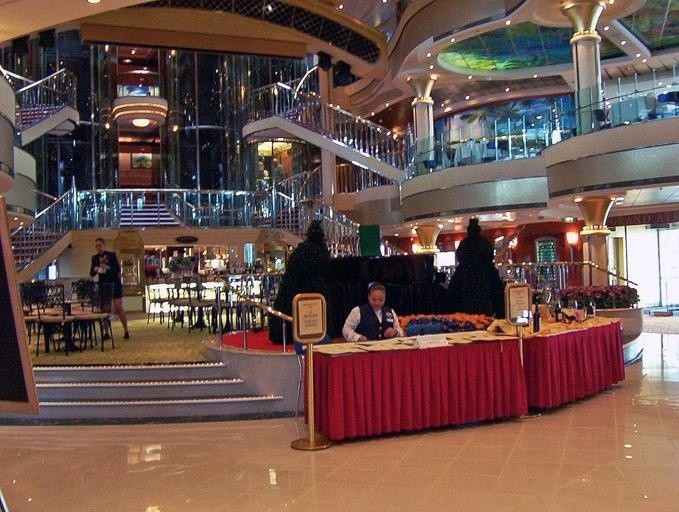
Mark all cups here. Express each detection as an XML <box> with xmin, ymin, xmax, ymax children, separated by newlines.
<box><xmin>63</xmin><ymin>303</ymin><xmax>71</xmax><ymax>316</ymax></box>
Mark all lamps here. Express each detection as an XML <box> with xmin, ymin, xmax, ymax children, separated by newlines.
<box><xmin>110</xmin><ymin>98</ymin><xmax>168</xmax><ymax>132</ymax></box>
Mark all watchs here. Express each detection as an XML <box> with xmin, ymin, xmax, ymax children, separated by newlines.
<box><xmin>394</xmin><ymin>329</ymin><xmax>398</xmax><ymax>337</ymax></box>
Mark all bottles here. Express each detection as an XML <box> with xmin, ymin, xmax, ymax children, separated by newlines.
<box><xmin>533</xmin><ymin>303</ymin><xmax>541</xmax><ymax>332</ymax></box>
<box><xmin>587</xmin><ymin>297</ymin><xmax>594</xmax><ymax>317</ymax></box>
<box><xmin>80</xmin><ymin>303</ymin><xmax>84</xmax><ymax>313</ymax></box>
<box><xmin>573</xmin><ymin>294</ymin><xmax>578</xmax><ymax>310</ymax></box>
<box><xmin>556</xmin><ymin>297</ymin><xmax>562</xmax><ymax>322</ymax></box>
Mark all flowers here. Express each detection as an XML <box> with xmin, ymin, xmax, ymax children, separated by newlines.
<box><xmin>395</xmin><ymin>309</ymin><xmax>496</xmax><ymax>330</ymax></box>
<box><xmin>561</xmin><ymin>281</ymin><xmax>639</xmax><ymax>305</ymax></box>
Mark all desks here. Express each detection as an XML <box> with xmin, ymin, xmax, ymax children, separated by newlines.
<box><xmin>304</xmin><ymin>328</ymin><xmax>525</xmax><ymax>448</ymax></box>
<box><xmin>517</xmin><ymin>310</ymin><xmax>625</xmax><ymax>408</ymax></box>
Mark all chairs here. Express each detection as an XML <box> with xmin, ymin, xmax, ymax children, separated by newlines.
<box><xmin>144</xmin><ymin>272</ymin><xmax>278</xmax><ymax>336</ymax></box>
<box><xmin>20</xmin><ymin>276</ymin><xmax>115</xmax><ymax>358</ymax></box>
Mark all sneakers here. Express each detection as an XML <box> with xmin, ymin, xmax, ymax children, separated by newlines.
<box><xmin>103</xmin><ymin>333</ymin><xmax>129</xmax><ymax>340</ymax></box>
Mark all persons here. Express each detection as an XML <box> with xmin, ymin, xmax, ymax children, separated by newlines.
<box><xmin>89</xmin><ymin>237</ymin><xmax>130</xmax><ymax>341</ymax></box>
<box><xmin>341</xmin><ymin>281</ymin><xmax>403</xmax><ymax>342</ymax></box>
<box><xmin>271</xmin><ymin>158</ymin><xmax>285</xmax><ymax>210</ymax></box>
<box><xmin>169</xmin><ymin>249</ymin><xmax>185</xmax><ymax>277</ymax></box>
